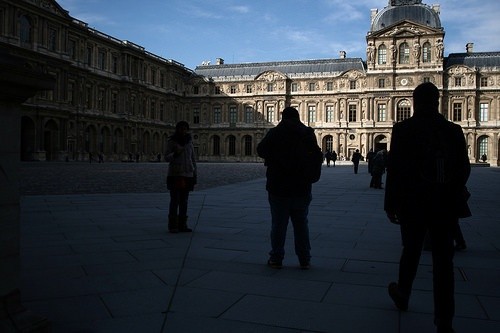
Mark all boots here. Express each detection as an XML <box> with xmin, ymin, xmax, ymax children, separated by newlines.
<box><xmin>177</xmin><ymin>215</ymin><xmax>193</xmax><ymax>232</ymax></box>
<box><xmin>167</xmin><ymin>213</ymin><xmax>179</xmax><ymax>233</ymax></box>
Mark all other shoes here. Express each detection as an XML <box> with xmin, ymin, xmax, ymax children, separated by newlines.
<box><xmin>436</xmin><ymin>328</ymin><xmax>454</xmax><ymax>333</ymax></box>
<box><xmin>300</xmin><ymin>257</ymin><xmax>311</xmax><ymax>269</ymax></box>
<box><xmin>389</xmin><ymin>282</ymin><xmax>408</xmax><ymax>310</ymax></box>
<box><xmin>455</xmin><ymin>245</ymin><xmax>462</xmax><ymax>251</ymax></box>
<box><xmin>265</xmin><ymin>257</ymin><xmax>281</xmax><ymax>269</ymax></box>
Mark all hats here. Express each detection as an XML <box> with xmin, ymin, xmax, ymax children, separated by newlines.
<box><xmin>281</xmin><ymin>107</ymin><xmax>298</xmax><ymax>117</ymax></box>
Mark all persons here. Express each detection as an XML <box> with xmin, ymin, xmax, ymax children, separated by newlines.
<box><xmin>384</xmin><ymin>82</ymin><xmax>470</xmax><ymax>333</ymax></box>
<box><xmin>321</xmin><ymin>146</ymin><xmax>467</xmax><ymax>251</ymax></box>
<box><xmin>88</xmin><ymin>152</ymin><xmax>171</xmax><ymax>164</ymax></box>
<box><xmin>165</xmin><ymin>121</ymin><xmax>197</xmax><ymax>234</ymax></box>
<box><xmin>258</xmin><ymin>107</ymin><xmax>322</xmax><ymax>270</ymax></box>
